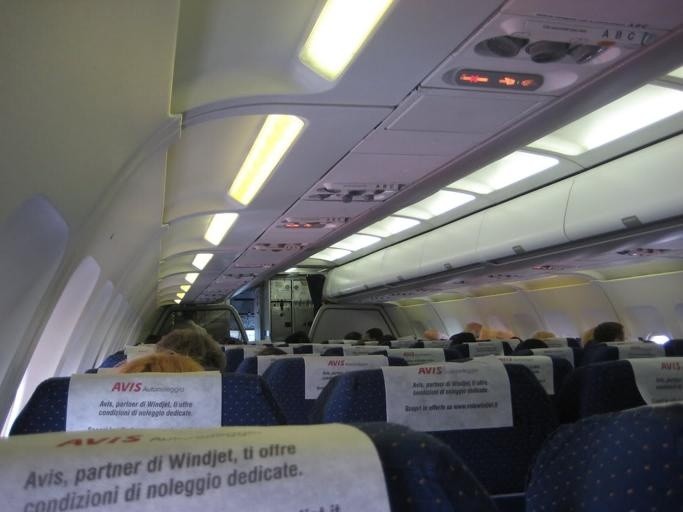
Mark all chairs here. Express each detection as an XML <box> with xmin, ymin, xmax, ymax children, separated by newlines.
<box><xmin>523</xmin><ymin>399</ymin><xmax>682</xmax><ymax>511</ymax></box>
<box><xmin>1</xmin><ymin>419</ymin><xmax>501</xmax><ymax>510</ymax></box>
<box><xmin>9</xmin><ymin>371</ymin><xmax>289</xmax><ymax>437</ymax></box>
<box><xmin>92</xmin><ymin>332</ymin><xmax>683</xmax><ymax>415</ymax></box>
<box><xmin>574</xmin><ymin>356</ymin><xmax>683</xmax><ymax>420</ymax></box>
<box><xmin>309</xmin><ymin>353</ymin><xmax>561</xmax><ymax>511</ymax></box>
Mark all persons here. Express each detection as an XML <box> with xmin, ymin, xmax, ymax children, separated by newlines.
<box><xmin>345</xmin><ymin>328</ymin><xmax>398</xmax><ymax>347</ymax></box>
<box><xmin>425</xmin><ymin>322</ymin><xmax>623</xmax><ymax>351</ymax></box>
<box><xmin>121</xmin><ymin>325</ymin><xmax>309</xmax><ymax>373</ymax></box>
